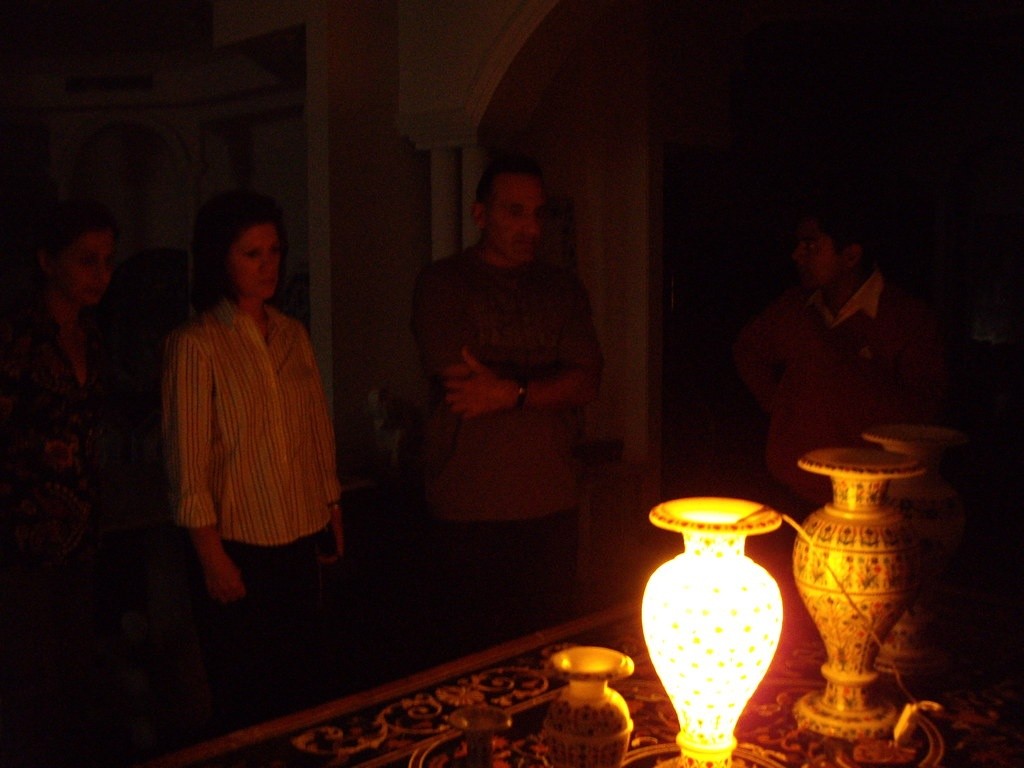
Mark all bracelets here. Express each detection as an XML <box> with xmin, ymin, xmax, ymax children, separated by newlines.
<box><xmin>511</xmin><ymin>368</ymin><xmax>530</xmax><ymax>412</ymax></box>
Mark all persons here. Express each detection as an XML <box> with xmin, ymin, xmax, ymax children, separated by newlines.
<box><xmin>730</xmin><ymin>194</ymin><xmax>954</xmax><ymax>564</ymax></box>
<box><xmin>0</xmin><ymin>199</ymin><xmax>120</xmax><ymax>768</ymax></box>
<box><xmin>393</xmin><ymin>150</ymin><xmax>606</xmax><ymax>675</ymax></box>
<box><xmin>157</xmin><ymin>190</ymin><xmax>345</xmax><ymax>735</ymax></box>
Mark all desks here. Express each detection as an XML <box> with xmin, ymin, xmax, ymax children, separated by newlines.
<box><xmin>146</xmin><ymin>570</ymin><xmax>1024</xmax><ymax>768</ymax></box>
